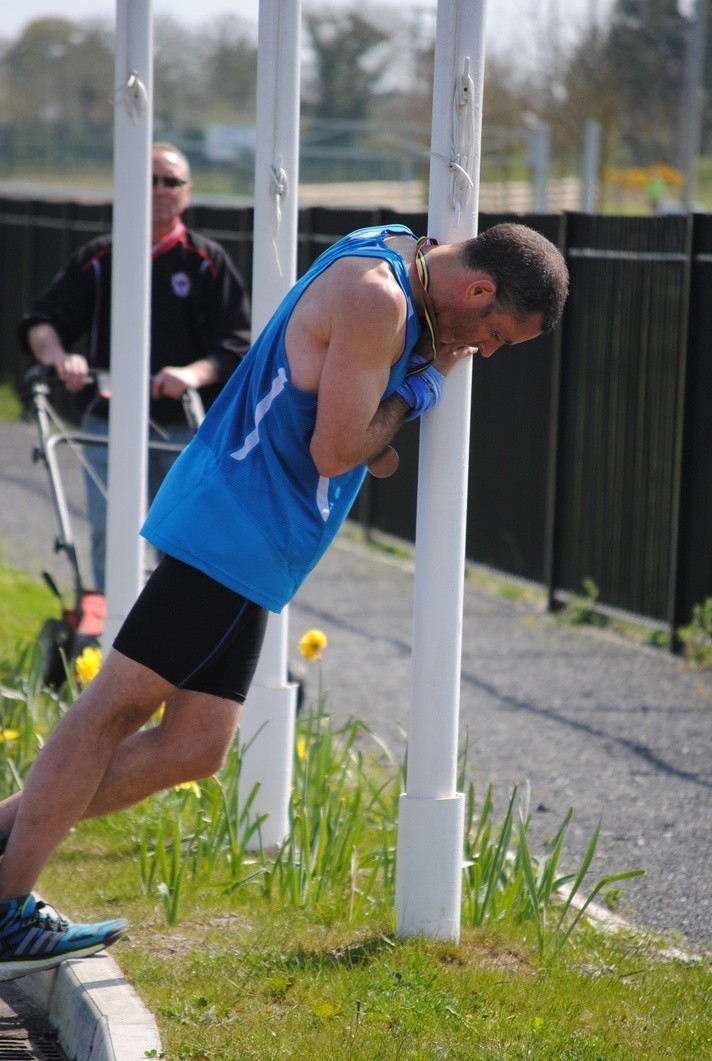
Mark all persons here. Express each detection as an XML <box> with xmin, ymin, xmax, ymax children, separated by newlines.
<box><xmin>1</xmin><ymin>225</ymin><xmax>570</xmax><ymax>982</ymax></box>
<box><xmin>18</xmin><ymin>141</ymin><xmax>250</xmax><ymax>594</ymax></box>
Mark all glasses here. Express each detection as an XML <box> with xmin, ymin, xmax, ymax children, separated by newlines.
<box><xmin>153</xmin><ymin>174</ymin><xmax>189</xmax><ymax>189</ymax></box>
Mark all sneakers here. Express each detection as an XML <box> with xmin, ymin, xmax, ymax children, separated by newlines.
<box><xmin>0</xmin><ymin>890</ymin><xmax>130</xmax><ymax>982</ymax></box>
